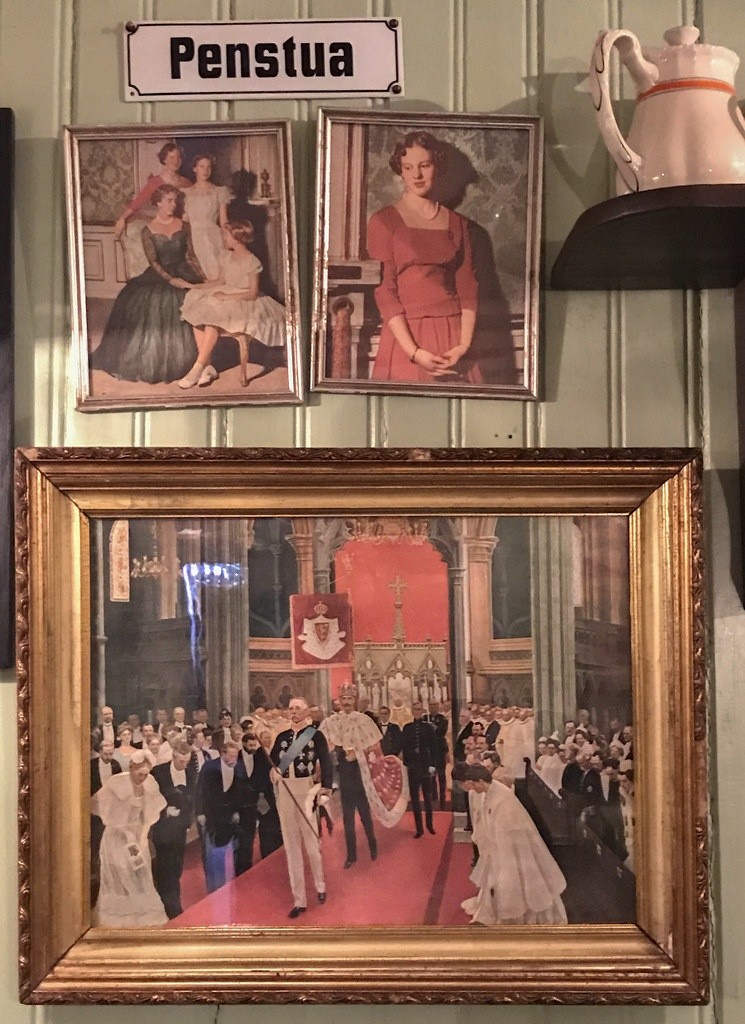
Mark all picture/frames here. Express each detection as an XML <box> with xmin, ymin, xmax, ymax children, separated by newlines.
<box><xmin>307</xmin><ymin>103</ymin><xmax>547</xmax><ymax>400</ymax></box>
<box><xmin>13</xmin><ymin>445</ymin><xmax>711</xmax><ymax>1007</ymax></box>
<box><xmin>62</xmin><ymin>118</ymin><xmax>303</xmax><ymax>412</ymax></box>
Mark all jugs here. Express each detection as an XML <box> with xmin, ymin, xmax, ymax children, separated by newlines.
<box><xmin>586</xmin><ymin>21</ymin><xmax>745</xmax><ymax>201</ymax></box>
